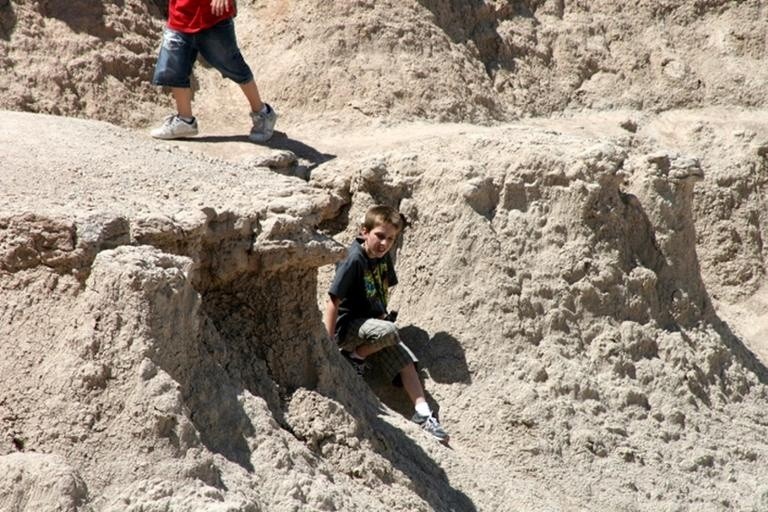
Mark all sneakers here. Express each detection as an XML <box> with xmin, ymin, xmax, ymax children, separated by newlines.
<box><xmin>249</xmin><ymin>102</ymin><xmax>277</xmax><ymax>143</ymax></box>
<box><xmin>342</xmin><ymin>350</ymin><xmax>369</xmax><ymax>379</ymax></box>
<box><xmin>151</xmin><ymin>113</ymin><xmax>199</xmax><ymax>140</ymax></box>
<box><xmin>411</xmin><ymin>410</ymin><xmax>450</xmax><ymax>442</ymax></box>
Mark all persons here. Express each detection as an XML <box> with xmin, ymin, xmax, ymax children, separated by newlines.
<box><xmin>325</xmin><ymin>203</ymin><xmax>450</xmax><ymax>445</ymax></box>
<box><xmin>150</xmin><ymin>0</ymin><xmax>278</xmax><ymax>144</ymax></box>
